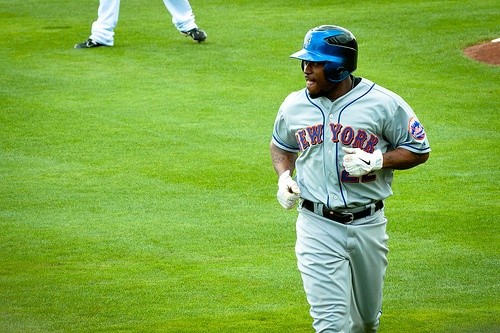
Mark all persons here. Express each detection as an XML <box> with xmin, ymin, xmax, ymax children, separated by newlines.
<box><xmin>269</xmin><ymin>25</ymin><xmax>431</xmax><ymax>333</ymax></box>
<box><xmin>73</xmin><ymin>0</ymin><xmax>208</xmax><ymax>48</ymax></box>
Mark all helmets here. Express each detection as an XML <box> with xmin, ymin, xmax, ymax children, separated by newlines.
<box><xmin>289</xmin><ymin>25</ymin><xmax>359</xmax><ymax>83</ymax></box>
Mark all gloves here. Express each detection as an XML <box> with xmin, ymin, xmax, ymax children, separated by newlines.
<box><xmin>342</xmin><ymin>145</ymin><xmax>384</xmax><ymax>176</ymax></box>
<box><xmin>276</xmin><ymin>169</ymin><xmax>300</xmax><ymax>210</ymax></box>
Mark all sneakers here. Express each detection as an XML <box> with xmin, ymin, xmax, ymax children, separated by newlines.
<box><xmin>74</xmin><ymin>39</ymin><xmax>105</xmax><ymax>49</ymax></box>
<box><xmin>180</xmin><ymin>29</ymin><xmax>208</xmax><ymax>42</ymax></box>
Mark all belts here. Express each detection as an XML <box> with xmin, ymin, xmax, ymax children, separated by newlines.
<box><xmin>299</xmin><ymin>199</ymin><xmax>384</xmax><ymax>224</ymax></box>
